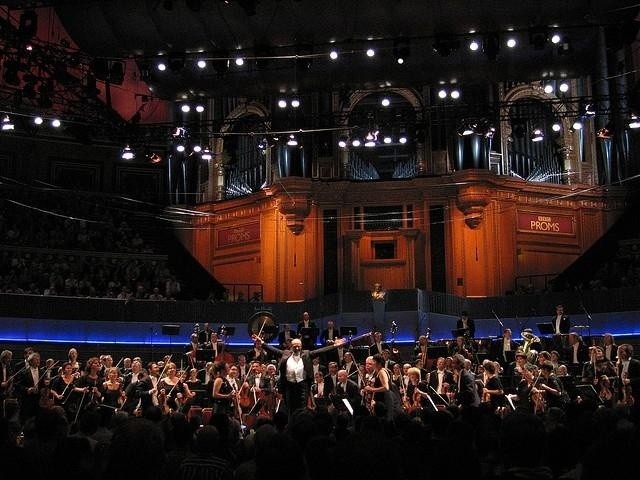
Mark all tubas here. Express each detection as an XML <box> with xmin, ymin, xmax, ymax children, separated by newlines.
<box><xmin>515</xmin><ymin>331</ymin><xmax>541</xmax><ymax>364</ymax></box>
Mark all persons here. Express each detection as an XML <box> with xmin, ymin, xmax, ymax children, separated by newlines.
<box><xmin>457</xmin><ymin>311</ymin><xmax>475</xmax><ymax>339</ymax></box>
<box><xmin>1</xmin><ymin>181</ymin><xmax>180</xmax><ymax>301</ymax></box>
<box><xmin>297</xmin><ymin>312</ymin><xmax>317</xmax><ymax>337</ymax></box>
<box><xmin>1</xmin><ymin>322</ymin><xmax>639</xmax><ymax>479</ymax></box>
<box><xmin>371</xmin><ymin>283</ymin><xmax>385</xmax><ymax>303</ymax></box>
<box><xmin>552</xmin><ymin>305</ymin><xmax>570</xmax><ymax>334</ymax></box>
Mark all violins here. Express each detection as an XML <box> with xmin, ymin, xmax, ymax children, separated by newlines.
<box><xmin>532</xmin><ymin>393</ymin><xmax>548</xmax><ymax>416</ymax></box>
<box><xmin>364</xmin><ymin>370</ymin><xmax>376</xmax><ymax>408</ymax></box>
<box><xmin>158</xmin><ymin>380</ymin><xmax>170</xmax><ymax>416</ymax></box>
<box><xmin>233</xmin><ymin>383</ymin><xmax>242</xmax><ymax>419</ymax></box>
